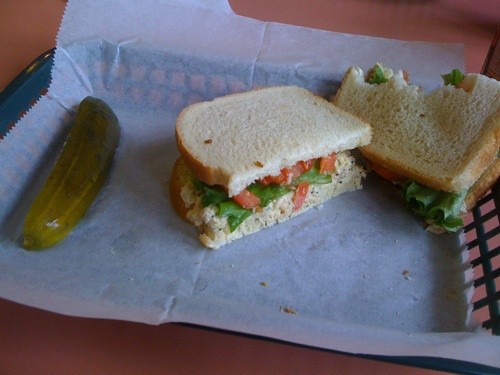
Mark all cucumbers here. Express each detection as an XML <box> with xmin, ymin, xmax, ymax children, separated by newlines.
<box><xmin>21</xmin><ymin>96</ymin><xmax>120</xmax><ymax>250</ymax></box>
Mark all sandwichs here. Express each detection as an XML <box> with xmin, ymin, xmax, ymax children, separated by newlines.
<box><xmin>169</xmin><ymin>84</ymin><xmax>373</xmax><ymax>249</ymax></box>
<box><xmin>330</xmin><ymin>62</ymin><xmax>500</xmax><ymax>234</ymax></box>
